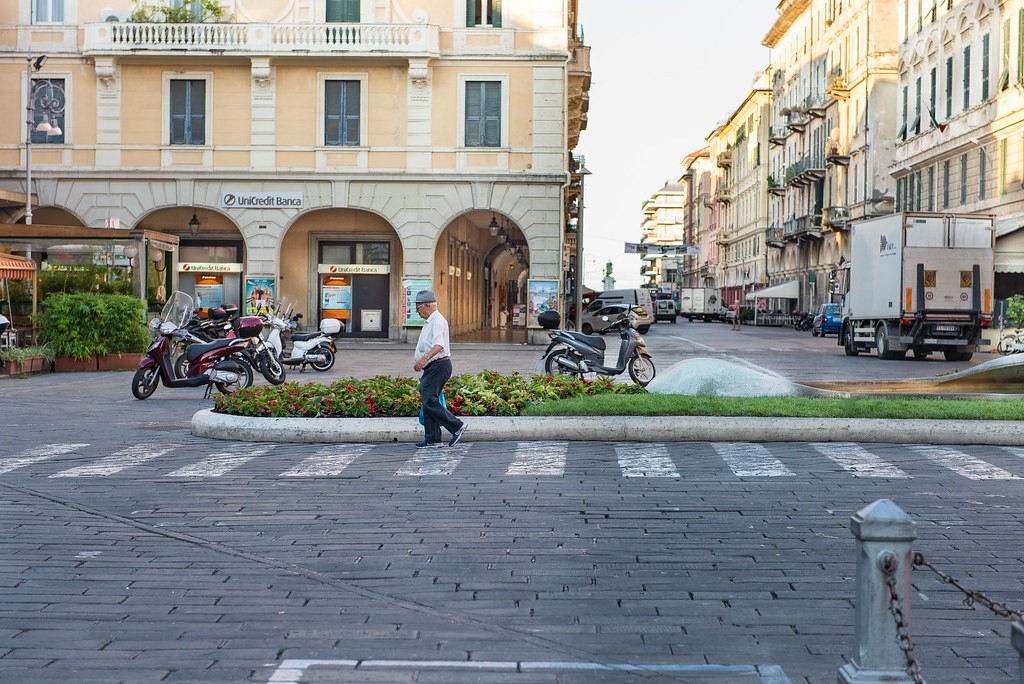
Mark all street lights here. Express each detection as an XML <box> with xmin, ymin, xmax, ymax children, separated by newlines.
<box><xmin>23</xmin><ymin>79</ymin><xmax>66</xmax><ymax>260</ymax></box>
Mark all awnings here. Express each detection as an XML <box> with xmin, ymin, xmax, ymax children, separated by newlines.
<box><xmin>996</xmin><ymin>215</ymin><xmax>1024</xmax><ymax>273</ymax></box>
<box><xmin>746</xmin><ymin>281</ymin><xmax>800</xmax><ymax>325</ymax></box>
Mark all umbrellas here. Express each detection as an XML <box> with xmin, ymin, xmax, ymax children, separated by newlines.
<box><xmin>155</xmin><ymin>250</ymin><xmax>166</xmax><ymax>303</ymax></box>
<box><xmin>0</xmin><ymin>257</ymin><xmax>33</xmax><ymax>280</ymax></box>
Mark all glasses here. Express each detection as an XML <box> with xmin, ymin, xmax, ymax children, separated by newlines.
<box><xmin>415</xmin><ymin>304</ymin><xmax>426</xmax><ymax>309</ymax></box>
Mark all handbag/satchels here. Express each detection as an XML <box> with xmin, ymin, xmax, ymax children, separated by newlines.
<box><xmin>419</xmin><ymin>390</ymin><xmax>448</xmax><ymax>425</ymax></box>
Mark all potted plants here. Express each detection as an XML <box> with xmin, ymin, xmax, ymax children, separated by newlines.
<box><xmin>0</xmin><ymin>336</ymin><xmax>58</xmax><ymax>379</ymax></box>
<box><xmin>27</xmin><ymin>291</ymin><xmax>151</xmax><ymax>371</ymax></box>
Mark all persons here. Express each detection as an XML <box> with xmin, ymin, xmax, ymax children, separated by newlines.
<box><xmin>731</xmin><ymin>301</ymin><xmax>741</xmax><ymax>330</ymax></box>
<box><xmin>414</xmin><ymin>291</ymin><xmax>468</xmax><ymax>448</ymax></box>
<box><xmin>251</xmin><ymin>284</ymin><xmax>272</xmax><ymax>307</ymax></box>
<box><xmin>0</xmin><ymin>315</ymin><xmax>10</xmax><ymax>335</ymax></box>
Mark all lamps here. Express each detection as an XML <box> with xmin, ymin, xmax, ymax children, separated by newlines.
<box><xmin>123</xmin><ymin>245</ymin><xmax>140</xmax><ymax>269</ymax></box>
<box><xmin>189</xmin><ymin>209</ymin><xmax>200</xmax><ymax>238</ymax></box>
<box><xmin>969</xmin><ymin>137</ymin><xmax>993</xmax><ymax>169</ymax></box>
<box><xmin>939</xmin><ymin>121</ymin><xmax>951</xmax><ymax>133</ymax></box>
<box><xmin>151</xmin><ymin>250</ymin><xmax>165</xmax><ymax>272</ymax></box>
<box><xmin>488</xmin><ymin>212</ymin><xmax>499</xmax><ymax>236</ymax></box>
<box><xmin>461</xmin><ymin>242</ymin><xmax>468</xmax><ymax>250</ymax></box>
<box><xmin>904</xmin><ymin>165</ymin><xmax>924</xmax><ymax>190</ymax></box>
<box><xmin>505</xmin><ymin>220</ymin><xmax>529</xmax><ymax>268</ymax></box>
<box><xmin>497</xmin><ymin>214</ymin><xmax>507</xmax><ymax>243</ymax></box>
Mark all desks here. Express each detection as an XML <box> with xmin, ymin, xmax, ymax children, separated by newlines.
<box><xmin>15</xmin><ymin>322</ymin><xmax>39</xmax><ymax>347</ymax></box>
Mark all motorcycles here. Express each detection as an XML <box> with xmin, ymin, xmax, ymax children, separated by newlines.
<box><xmin>131</xmin><ymin>290</ymin><xmax>264</xmax><ymax>400</ymax></box>
<box><xmin>537</xmin><ymin>303</ymin><xmax>656</xmax><ymax>386</ymax></box>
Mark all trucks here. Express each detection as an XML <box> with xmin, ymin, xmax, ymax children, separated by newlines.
<box><xmin>828</xmin><ymin>211</ymin><xmax>996</xmax><ymax>362</ymax></box>
<box><xmin>680</xmin><ymin>287</ymin><xmax>727</xmax><ymax>323</ymax></box>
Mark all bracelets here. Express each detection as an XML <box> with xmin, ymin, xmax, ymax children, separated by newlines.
<box><xmin>423</xmin><ymin>354</ymin><xmax>430</xmax><ymax>360</ymax></box>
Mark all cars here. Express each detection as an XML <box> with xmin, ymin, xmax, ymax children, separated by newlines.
<box><xmin>812</xmin><ymin>303</ymin><xmax>841</xmax><ymax>337</ymax></box>
<box><xmin>582</xmin><ymin>303</ymin><xmax>651</xmax><ymax>334</ymax></box>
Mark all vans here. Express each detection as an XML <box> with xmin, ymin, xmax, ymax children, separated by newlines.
<box><xmin>582</xmin><ymin>289</ymin><xmax>654</xmax><ymax>336</ymax></box>
<box><xmin>655</xmin><ymin>300</ymin><xmax>677</xmax><ymax>324</ymax></box>
<box><xmin>726</xmin><ymin>304</ymin><xmax>752</xmax><ymax>324</ymax></box>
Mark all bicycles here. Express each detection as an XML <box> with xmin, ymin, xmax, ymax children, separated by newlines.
<box><xmin>997</xmin><ymin>328</ymin><xmax>1024</xmax><ymax>356</ymax></box>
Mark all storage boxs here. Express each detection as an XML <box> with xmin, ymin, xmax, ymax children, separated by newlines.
<box><xmin>320</xmin><ymin>319</ymin><xmax>345</xmax><ymax>334</ymax></box>
<box><xmin>234</xmin><ymin>316</ymin><xmax>263</xmax><ymax>338</ymax></box>
<box><xmin>538</xmin><ymin>311</ymin><xmax>561</xmax><ymax>330</ymax></box>
<box><xmin>208</xmin><ymin>308</ymin><xmax>226</xmax><ymax>319</ymax></box>
<box><xmin>221</xmin><ymin>304</ymin><xmax>238</xmax><ymax>315</ymax></box>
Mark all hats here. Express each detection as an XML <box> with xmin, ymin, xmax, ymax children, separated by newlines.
<box><xmin>412</xmin><ymin>289</ymin><xmax>436</xmax><ymax>303</ymax></box>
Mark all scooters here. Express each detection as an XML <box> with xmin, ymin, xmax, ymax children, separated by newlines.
<box><xmin>155</xmin><ymin>302</ymin><xmax>346</xmax><ymax>384</ymax></box>
<box><xmin>793</xmin><ymin>309</ymin><xmax>816</xmax><ymax>331</ymax></box>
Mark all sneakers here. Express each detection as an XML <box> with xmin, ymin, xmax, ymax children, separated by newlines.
<box><xmin>449</xmin><ymin>424</ymin><xmax>467</xmax><ymax>447</ymax></box>
<box><xmin>415</xmin><ymin>439</ymin><xmax>445</xmax><ymax>448</ymax></box>
